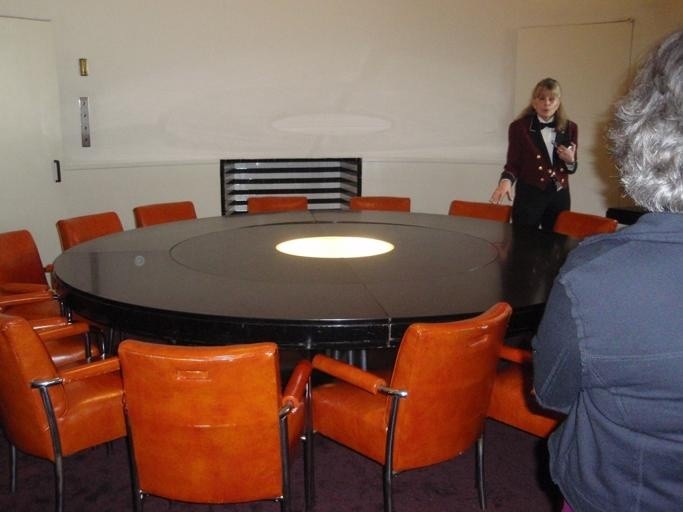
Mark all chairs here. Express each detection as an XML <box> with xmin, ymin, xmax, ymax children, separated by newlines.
<box><xmin>128</xmin><ymin>202</ymin><xmax>198</xmax><ymax>227</ymax></box>
<box><xmin>118</xmin><ymin>336</ymin><xmax>314</xmax><ymax>511</ymax></box>
<box><xmin>552</xmin><ymin>211</ymin><xmax>617</xmax><ymax>243</ymax></box>
<box><xmin>2</xmin><ymin>227</ymin><xmax>64</xmax><ymax>316</ymax></box>
<box><xmin>0</xmin><ymin>311</ymin><xmax>126</xmax><ymax>510</ymax></box>
<box><xmin>314</xmin><ymin>298</ymin><xmax>515</xmax><ymax>511</ymax></box>
<box><xmin>244</xmin><ymin>196</ymin><xmax>310</xmax><ymax>218</ymax></box>
<box><xmin>55</xmin><ymin>210</ymin><xmax>129</xmax><ymax>251</ymax></box>
<box><xmin>450</xmin><ymin>200</ymin><xmax>514</xmax><ymax>224</ymax></box>
<box><xmin>347</xmin><ymin>196</ymin><xmax>413</xmax><ymax>212</ymax></box>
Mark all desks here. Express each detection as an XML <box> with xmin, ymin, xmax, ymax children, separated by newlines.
<box><xmin>45</xmin><ymin>209</ymin><xmax>598</xmax><ymax>353</ymax></box>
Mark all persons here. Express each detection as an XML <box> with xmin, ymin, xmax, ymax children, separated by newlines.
<box><xmin>529</xmin><ymin>31</ymin><xmax>683</xmax><ymax>512</ymax></box>
<box><xmin>488</xmin><ymin>76</ymin><xmax>580</xmax><ymax>233</ymax></box>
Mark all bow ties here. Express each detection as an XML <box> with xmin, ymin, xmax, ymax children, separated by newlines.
<box><xmin>539</xmin><ymin>122</ymin><xmax>556</xmax><ymax>129</ymax></box>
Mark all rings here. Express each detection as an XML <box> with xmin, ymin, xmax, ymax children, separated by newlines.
<box><xmin>558</xmin><ymin>150</ymin><xmax>563</xmax><ymax>153</ymax></box>
